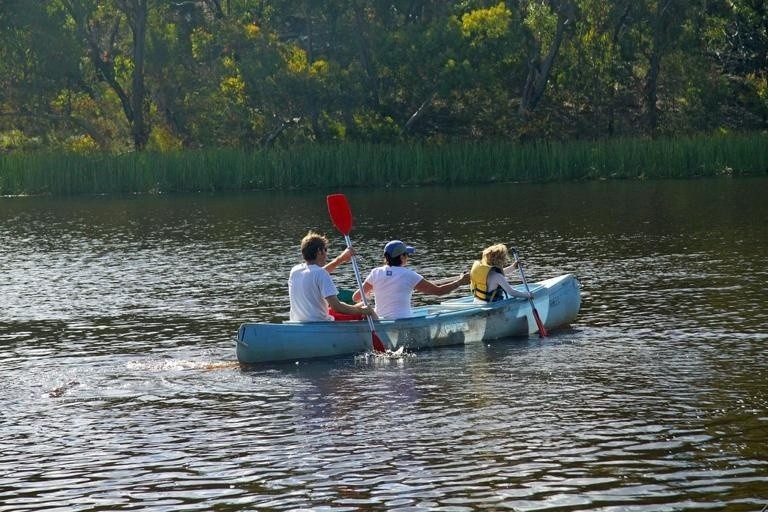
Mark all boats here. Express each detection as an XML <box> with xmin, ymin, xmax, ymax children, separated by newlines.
<box><xmin>237</xmin><ymin>271</ymin><xmax>581</xmax><ymax>367</ymax></box>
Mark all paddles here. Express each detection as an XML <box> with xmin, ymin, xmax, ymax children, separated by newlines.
<box><xmin>326</xmin><ymin>193</ymin><xmax>386</xmax><ymax>352</ymax></box>
<box><xmin>513</xmin><ymin>252</ymin><xmax>549</xmax><ymax>338</ymax></box>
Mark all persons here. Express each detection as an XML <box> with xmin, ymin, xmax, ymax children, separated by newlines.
<box><xmin>470</xmin><ymin>243</ymin><xmax>534</xmax><ymax>305</ymax></box>
<box><xmin>288</xmin><ymin>233</ymin><xmax>371</xmax><ymax>322</ymax></box>
<box><xmin>351</xmin><ymin>239</ymin><xmax>472</xmax><ymax>319</ymax></box>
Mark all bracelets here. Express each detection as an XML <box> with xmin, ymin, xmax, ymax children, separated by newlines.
<box><xmin>337</xmin><ymin>256</ymin><xmax>343</xmax><ymax>263</ymax></box>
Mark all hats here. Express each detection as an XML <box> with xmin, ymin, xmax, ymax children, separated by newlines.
<box><xmin>383</xmin><ymin>240</ymin><xmax>416</xmax><ymax>258</ymax></box>
